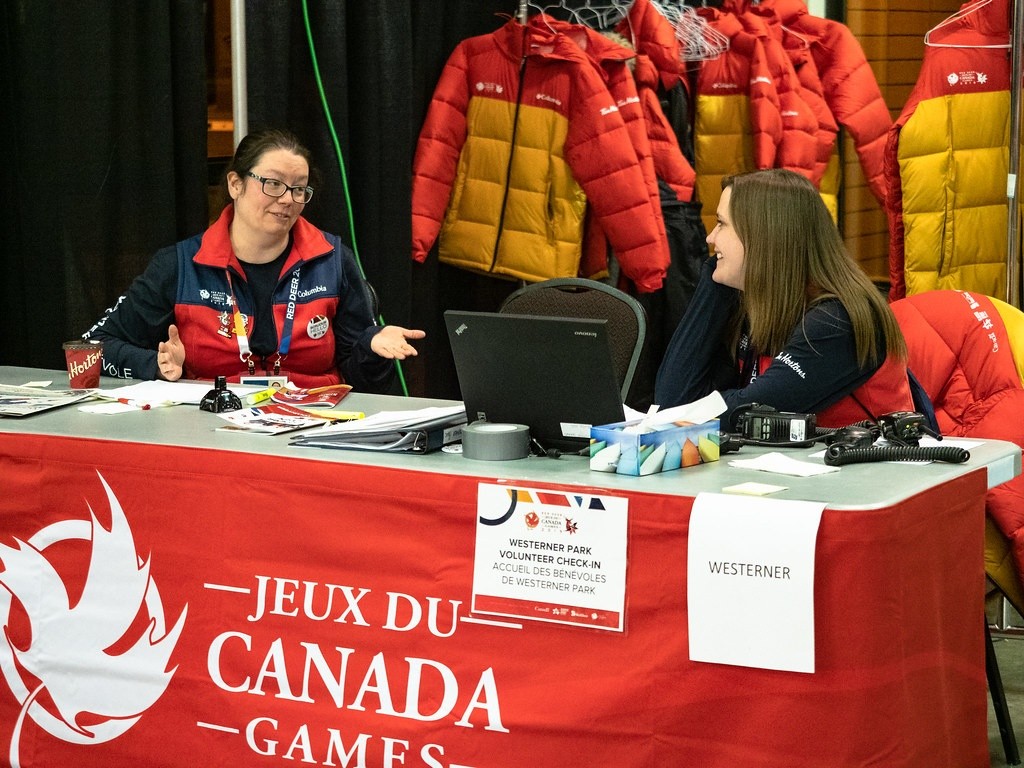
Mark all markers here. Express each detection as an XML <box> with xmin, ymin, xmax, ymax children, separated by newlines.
<box><xmin>247</xmin><ymin>387</ymin><xmax>278</xmax><ymax>404</ymax></box>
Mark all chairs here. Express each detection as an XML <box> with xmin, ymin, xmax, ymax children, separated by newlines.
<box><xmin>495</xmin><ymin>276</ymin><xmax>650</xmax><ymax>403</ymax></box>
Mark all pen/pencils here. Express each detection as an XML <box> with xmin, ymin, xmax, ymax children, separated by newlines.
<box><xmin>118</xmin><ymin>397</ymin><xmax>151</xmax><ymax>410</ymax></box>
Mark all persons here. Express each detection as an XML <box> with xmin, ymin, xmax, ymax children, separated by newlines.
<box><xmin>78</xmin><ymin>126</ymin><xmax>426</xmax><ymax>389</ymax></box>
<box><xmin>654</xmin><ymin>167</ymin><xmax>919</xmax><ymax>430</ymax></box>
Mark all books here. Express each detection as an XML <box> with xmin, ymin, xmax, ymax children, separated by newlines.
<box><xmin>0</xmin><ymin>383</ymin><xmax>102</xmax><ymax>418</ymax></box>
<box><xmin>214</xmin><ymin>403</ymin><xmax>339</xmax><ymax>436</ymax></box>
<box><xmin>266</xmin><ymin>381</ymin><xmax>353</xmax><ymax>409</ymax></box>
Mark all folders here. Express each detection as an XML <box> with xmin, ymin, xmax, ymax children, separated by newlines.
<box><xmin>289</xmin><ymin>408</ymin><xmax>468</xmax><ymax>456</ymax></box>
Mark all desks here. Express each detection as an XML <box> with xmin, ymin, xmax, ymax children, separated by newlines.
<box><xmin>0</xmin><ymin>362</ymin><xmax>1021</xmax><ymax>768</ymax></box>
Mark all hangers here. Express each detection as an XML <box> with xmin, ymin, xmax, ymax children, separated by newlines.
<box><xmin>525</xmin><ymin>0</ymin><xmax>730</xmax><ymax>61</ymax></box>
<box><xmin>923</xmin><ymin>0</ymin><xmax>1013</xmax><ymax>49</ymax></box>
<box><xmin>779</xmin><ymin>26</ymin><xmax>810</xmax><ymax>53</ymax></box>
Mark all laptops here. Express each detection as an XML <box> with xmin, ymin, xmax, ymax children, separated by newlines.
<box><xmin>445</xmin><ymin>310</ymin><xmax>626</xmax><ymax>453</ymax></box>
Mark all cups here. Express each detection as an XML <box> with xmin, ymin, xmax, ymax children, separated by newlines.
<box><xmin>62</xmin><ymin>340</ymin><xmax>103</xmax><ymax>389</ymax></box>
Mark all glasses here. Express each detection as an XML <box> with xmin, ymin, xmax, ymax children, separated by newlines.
<box><xmin>247</xmin><ymin>172</ymin><xmax>314</xmax><ymax>204</ymax></box>
